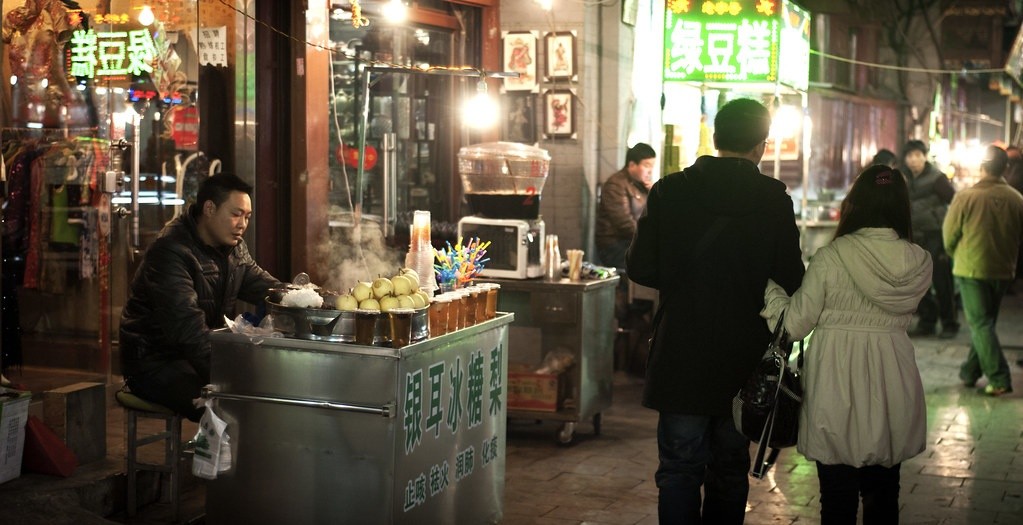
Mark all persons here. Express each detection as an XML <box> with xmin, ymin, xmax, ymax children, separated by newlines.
<box><xmin>119</xmin><ymin>172</ymin><xmax>288</xmax><ymax>423</ymax></box>
<box><xmin>758</xmin><ymin>164</ymin><xmax>935</xmax><ymax>525</ymax></box>
<box><xmin>625</xmin><ymin>99</ymin><xmax>806</xmax><ymax>525</ymax></box>
<box><xmin>592</xmin><ymin>142</ymin><xmax>655</xmax><ymax>262</ymax></box>
<box><xmin>939</xmin><ymin>145</ymin><xmax>1023</xmax><ymax>395</ymax></box>
<box><xmin>872</xmin><ymin>139</ymin><xmax>1023</xmax><ymax>341</ymax></box>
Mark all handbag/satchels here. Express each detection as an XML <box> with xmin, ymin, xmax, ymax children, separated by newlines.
<box><xmin>731</xmin><ymin>306</ymin><xmax>806</xmax><ymax>480</ymax></box>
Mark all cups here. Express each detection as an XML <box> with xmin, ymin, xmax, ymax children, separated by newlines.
<box><xmin>404</xmin><ymin>210</ymin><xmax>502</xmax><ymax>337</ymax></box>
<box><xmin>352</xmin><ymin>307</ymin><xmax>381</xmax><ymax>347</ymax></box>
<box><xmin>543</xmin><ymin>234</ymin><xmax>563</xmax><ymax>284</ymax></box>
<box><xmin>387</xmin><ymin>307</ymin><xmax>415</xmax><ymax>349</ymax></box>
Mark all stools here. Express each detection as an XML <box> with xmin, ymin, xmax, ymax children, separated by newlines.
<box><xmin>116</xmin><ymin>390</ymin><xmax>207</xmax><ymax>525</ymax></box>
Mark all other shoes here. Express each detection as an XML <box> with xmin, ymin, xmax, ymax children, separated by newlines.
<box><xmin>976</xmin><ymin>382</ymin><xmax>1013</xmax><ymax>396</ymax></box>
<box><xmin>940</xmin><ymin>320</ymin><xmax>960</xmax><ymax>338</ymax></box>
<box><xmin>912</xmin><ymin>315</ymin><xmax>939</xmax><ymax>336</ymax></box>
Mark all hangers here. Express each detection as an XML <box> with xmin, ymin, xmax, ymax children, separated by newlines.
<box><xmin>8</xmin><ymin>128</ymin><xmax>94</xmax><ymax>150</ymax></box>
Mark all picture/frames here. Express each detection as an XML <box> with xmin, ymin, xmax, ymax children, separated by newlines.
<box><xmin>501</xmin><ymin>29</ymin><xmax>578</xmax><ymax>143</ymax></box>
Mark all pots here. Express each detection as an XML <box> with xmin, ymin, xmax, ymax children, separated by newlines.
<box><xmin>264</xmin><ymin>294</ymin><xmax>430</xmax><ymax>349</ymax></box>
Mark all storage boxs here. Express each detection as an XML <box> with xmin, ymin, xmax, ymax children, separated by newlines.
<box><xmin>0</xmin><ymin>387</ymin><xmax>34</xmax><ymax>485</ymax></box>
<box><xmin>42</xmin><ymin>380</ymin><xmax>106</xmax><ymax>466</ymax></box>
<box><xmin>507</xmin><ymin>360</ymin><xmax>561</xmax><ymax>414</ymax></box>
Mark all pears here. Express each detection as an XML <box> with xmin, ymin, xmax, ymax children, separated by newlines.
<box><xmin>335</xmin><ymin>267</ymin><xmax>430</xmax><ymax>311</ymax></box>
